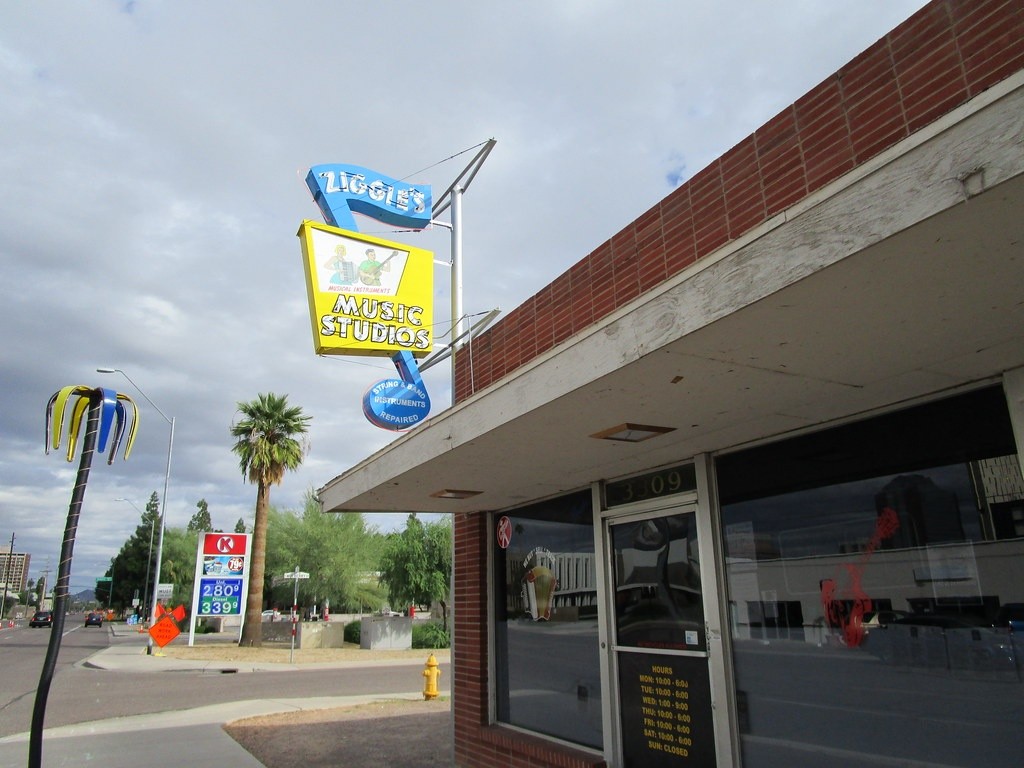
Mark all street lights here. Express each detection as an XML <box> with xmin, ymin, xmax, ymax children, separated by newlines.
<box><xmin>114</xmin><ymin>496</ymin><xmax>157</xmax><ymax>625</ymax></box>
<box><xmin>95</xmin><ymin>367</ymin><xmax>176</xmax><ymax>657</ymax></box>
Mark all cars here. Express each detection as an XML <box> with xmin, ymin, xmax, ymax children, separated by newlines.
<box><xmin>845</xmin><ymin>601</ymin><xmax>1023</xmax><ymax>669</ymax></box>
<box><xmin>29</xmin><ymin>610</ymin><xmax>53</xmax><ymax>629</ymax></box>
<box><xmin>84</xmin><ymin>611</ymin><xmax>105</xmax><ymax>628</ymax></box>
<box><xmin>604</xmin><ymin>578</ymin><xmax>831</xmax><ymax>704</ymax></box>
<box><xmin>261</xmin><ymin>609</ymin><xmax>281</xmax><ymax>618</ymax></box>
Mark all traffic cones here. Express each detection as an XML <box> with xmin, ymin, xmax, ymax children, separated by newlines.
<box><xmin>139</xmin><ymin>623</ymin><xmax>144</xmax><ymax>634</ymax></box>
<box><xmin>0</xmin><ymin>620</ymin><xmax>16</xmax><ymax>629</ymax></box>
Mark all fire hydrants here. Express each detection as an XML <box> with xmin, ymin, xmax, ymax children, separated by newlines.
<box><xmin>421</xmin><ymin>653</ymin><xmax>442</xmax><ymax>701</ymax></box>
